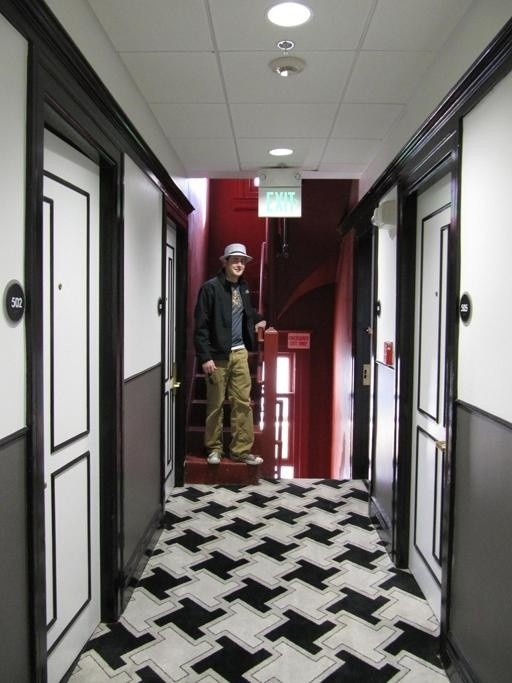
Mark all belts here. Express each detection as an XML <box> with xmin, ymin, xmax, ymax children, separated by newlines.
<box><xmin>230</xmin><ymin>343</ymin><xmax>245</xmax><ymax>350</ymax></box>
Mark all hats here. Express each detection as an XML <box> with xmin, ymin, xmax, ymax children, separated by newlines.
<box><xmin>218</xmin><ymin>243</ymin><xmax>253</xmax><ymax>262</ymax></box>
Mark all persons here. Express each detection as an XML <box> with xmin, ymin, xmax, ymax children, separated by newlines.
<box><xmin>194</xmin><ymin>243</ymin><xmax>266</xmax><ymax>465</ymax></box>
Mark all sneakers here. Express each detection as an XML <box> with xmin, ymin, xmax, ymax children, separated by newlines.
<box><xmin>207</xmin><ymin>451</ymin><xmax>223</xmax><ymax>464</ymax></box>
<box><xmin>230</xmin><ymin>452</ymin><xmax>264</xmax><ymax>465</ymax></box>
